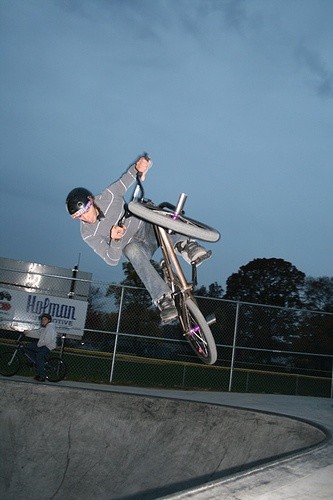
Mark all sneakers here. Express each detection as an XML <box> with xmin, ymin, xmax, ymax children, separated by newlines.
<box><xmin>182</xmin><ymin>242</ymin><xmax>206</xmax><ymax>266</ymax></box>
<box><xmin>153</xmin><ymin>295</ymin><xmax>179</xmax><ymax>321</ymax></box>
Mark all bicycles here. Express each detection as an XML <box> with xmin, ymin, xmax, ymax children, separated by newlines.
<box><xmin>0</xmin><ymin>323</ymin><xmax>67</xmax><ymax>383</ymax></box>
<box><xmin>106</xmin><ymin>152</ymin><xmax>223</xmax><ymax>366</ymax></box>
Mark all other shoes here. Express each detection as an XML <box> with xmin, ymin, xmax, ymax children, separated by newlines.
<box><xmin>34</xmin><ymin>374</ymin><xmax>46</xmax><ymax>382</ymax></box>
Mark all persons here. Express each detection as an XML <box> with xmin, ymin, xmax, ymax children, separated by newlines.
<box><xmin>64</xmin><ymin>155</ymin><xmax>207</xmax><ymax>321</ymax></box>
<box><xmin>22</xmin><ymin>313</ymin><xmax>56</xmax><ymax>381</ymax></box>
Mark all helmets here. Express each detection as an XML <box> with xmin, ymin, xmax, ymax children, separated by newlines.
<box><xmin>66</xmin><ymin>187</ymin><xmax>94</xmax><ymax>219</ymax></box>
<box><xmin>39</xmin><ymin>313</ymin><xmax>52</xmax><ymax>322</ymax></box>
